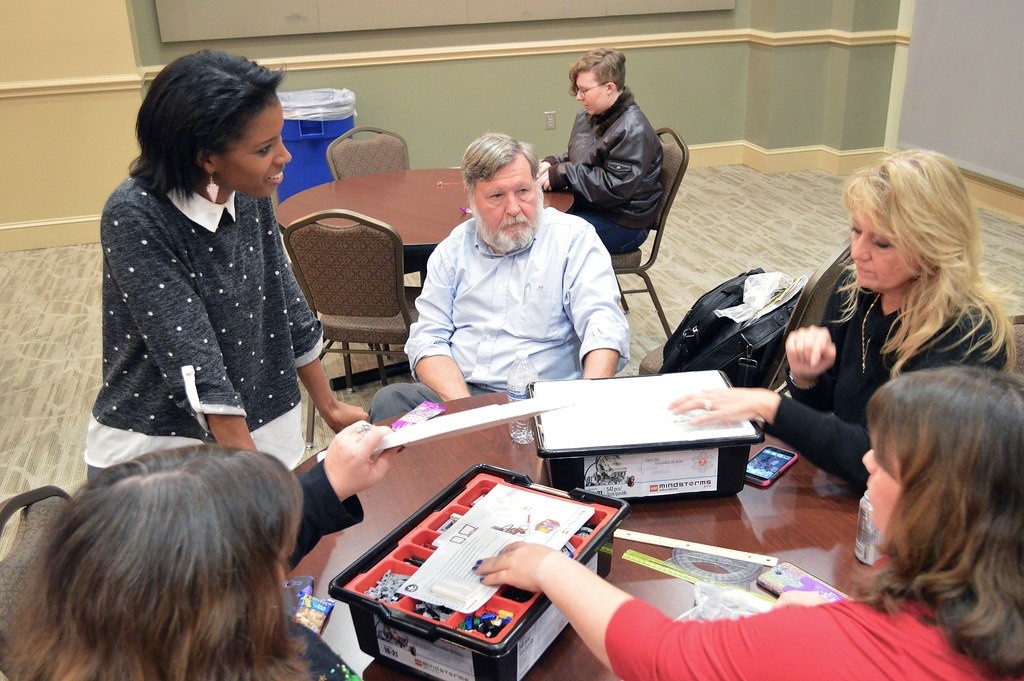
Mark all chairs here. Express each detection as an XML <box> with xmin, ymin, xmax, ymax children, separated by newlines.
<box><xmin>609</xmin><ymin>125</ymin><xmax>704</xmax><ymax>342</ymax></box>
<box><xmin>325</xmin><ymin>126</ymin><xmax>413</xmax><ymax>183</ymax></box>
<box><xmin>278</xmin><ymin>208</ymin><xmax>444</xmax><ymax>452</ymax></box>
<box><xmin>0</xmin><ymin>484</ymin><xmax>89</xmax><ymax>681</ymax></box>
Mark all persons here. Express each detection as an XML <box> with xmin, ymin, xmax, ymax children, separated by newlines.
<box><xmin>83</xmin><ymin>49</ymin><xmax>371</xmax><ymax>480</ymax></box>
<box><xmin>0</xmin><ymin>420</ymin><xmax>406</xmax><ymax>681</ymax></box>
<box><xmin>669</xmin><ymin>149</ymin><xmax>1017</xmax><ymax>486</ymax></box>
<box><xmin>537</xmin><ymin>48</ymin><xmax>665</xmax><ymax>253</ymax></box>
<box><xmin>367</xmin><ymin>133</ymin><xmax>631</xmax><ymax>423</ymax></box>
<box><xmin>472</xmin><ymin>366</ymin><xmax>1024</xmax><ymax>681</ymax></box>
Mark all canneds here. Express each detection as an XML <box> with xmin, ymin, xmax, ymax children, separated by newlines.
<box><xmin>854</xmin><ymin>490</ymin><xmax>885</xmax><ymax>566</ymax></box>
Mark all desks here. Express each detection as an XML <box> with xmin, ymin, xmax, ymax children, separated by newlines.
<box><xmin>288</xmin><ymin>390</ymin><xmax>1024</xmax><ymax>681</ymax></box>
<box><xmin>273</xmin><ymin>168</ymin><xmax>575</xmax><ymax>257</ymax></box>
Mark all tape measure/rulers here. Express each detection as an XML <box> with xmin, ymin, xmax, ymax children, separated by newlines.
<box><xmin>665</xmin><ymin>552</ymin><xmax>751</xmax><ymax>592</ymax></box>
<box><xmin>672</xmin><ymin>547</ymin><xmax>763</xmax><ymax>583</ymax></box>
<box><xmin>613</xmin><ymin>529</ymin><xmax>778</xmax><ymax>567</ymax></box>
<box><xmin>622</xmin><ymin>549</ymin><xmax>776</xmax><ymax>603</ymax></box>
<box><xmin>599</xmin><ymin>543</ymin><xmax>613</xmax><ymax>555</ymax></box>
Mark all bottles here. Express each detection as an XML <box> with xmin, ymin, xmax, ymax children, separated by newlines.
<box><xmin>506</xmin><ymin>351</ymin><xmax>538</xmax><ymax>445</ymax></box>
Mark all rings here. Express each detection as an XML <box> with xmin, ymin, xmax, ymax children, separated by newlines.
<box><xmin>356</xmin><ymin>424</ymin><xmax>371</xmax><ymax>436</ymax></box>
<box><xmin>704</xmin><ymin>399</ymin><xmax>711</xmax><ymax>411</ymax></box>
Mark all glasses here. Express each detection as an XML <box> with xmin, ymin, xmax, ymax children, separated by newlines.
<box><xmin>574</xmin><ymin>79</ymin><xmax>617</xmax><ymax>98</ymax></box>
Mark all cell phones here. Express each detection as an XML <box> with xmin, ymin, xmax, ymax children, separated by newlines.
<box><xmin>745</xmin><ymin>445</ymin><xmax>799</xmax><ymax>485</ymax></box>
<box><xmin>756</xmin><ymin>562</ymin><xmax>854</xmax><ymax>603</ymax></box>
<box><xmin>284</xmin><ymin>575</ymin><xmax>314</xmax><ymax>619</ymax></box>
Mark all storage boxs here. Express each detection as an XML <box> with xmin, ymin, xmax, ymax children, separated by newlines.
<box><xmin>534</xmin><ymin>369</ymin><xmax>765</xmax><ymax>503</ymax></box>
<box><xmin>319</xmin><ymin>462</ymin><xmax>633</xmax><ymax>681</ymax></box>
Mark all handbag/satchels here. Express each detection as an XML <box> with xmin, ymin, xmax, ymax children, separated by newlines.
<box><xmin>660</xmin><ymin>267</ymin><xmax>812</xmax><ymax>387</ymax></box>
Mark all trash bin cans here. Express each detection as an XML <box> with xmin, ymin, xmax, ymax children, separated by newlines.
<box><xmin>277</xmin><ymin>88</ymin><xmax>354</xmax><ymax>205</ymax></box>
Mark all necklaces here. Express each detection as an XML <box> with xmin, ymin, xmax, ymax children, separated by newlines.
<box><xmin>862</xmin><ymin>293</ymin><xmax>880</xmax><ymax>372</ymax></box>
